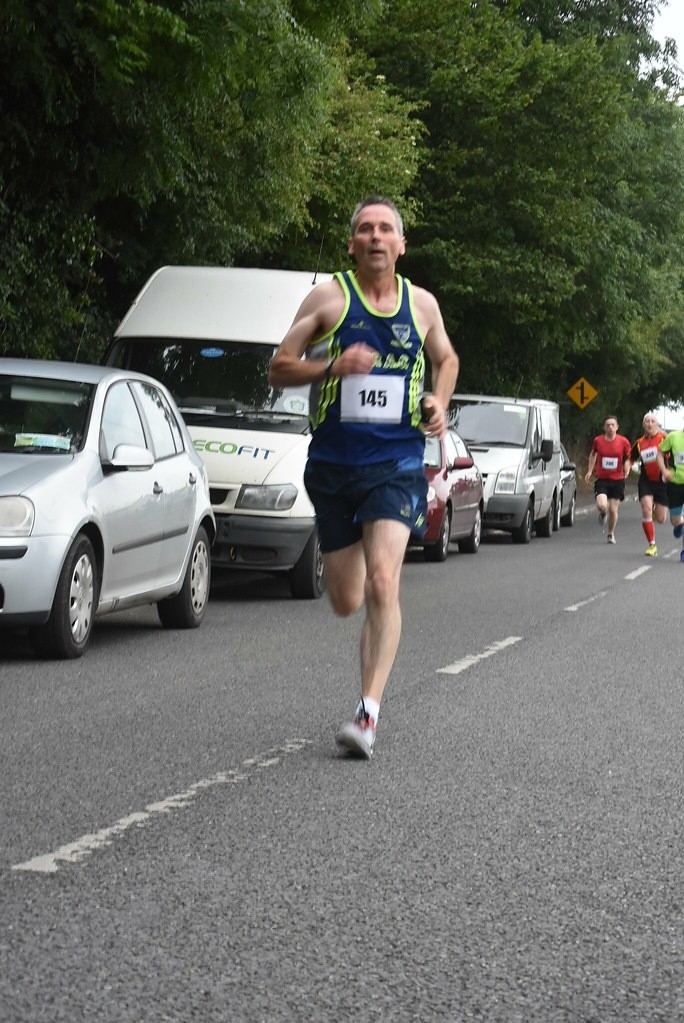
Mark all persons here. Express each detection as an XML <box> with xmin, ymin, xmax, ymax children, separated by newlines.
<box><xmin>584</xmin><ymin>415</ymin><xmax>632</xmax><ymax>544</ymax></box>
<box><xmin>657</xmin><ymin>428</ymin><xmax>684</xmax><ymax>562</ymax></box>
<box><xmin>624</xmin><ymin>412</ymin><xmax>669</xmax><ymax>557</ymax></box>
<box><xmin>267</xmin><ymin>194</ymin><xmax>460</xmax><ymax>759</ymax></box>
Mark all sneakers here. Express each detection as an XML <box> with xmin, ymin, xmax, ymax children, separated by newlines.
<box><xmin>598</xmin><ymin>513</ymin><xmax>609</xmax><ymax>527</ymax></box>
<box><xmin>605</xmin><ymin>533</ymin><xmax>615</xmax><ymax>545</ymax></box>
<box><xmin>338</xmin><ymin>712</ymin><xmax>380</xmax><ymax>760</ymax></box>
<box><xmin>672</xmin><ymin>521</ymin><xmax>684</xmax><ymax>538</ymax></box>
<box><xmin>646</xmin><ymin>541</ymin><xmax>657</xmax><ymax>556</ymax></box>
<box><xmin>680</xmin><ymin>551</ymin><xmax>684</xmax><ymax>561</ymax></box>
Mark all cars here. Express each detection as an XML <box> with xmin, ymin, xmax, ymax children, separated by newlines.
<box><xmin>403</xmin><ymin>427</ymin><xmax>485</xmax><ymax>564</ymax></box>
<box><xmin>552</xmin><ymin>442</ymin><xmax>577</xmax><ymax>533</ymax></box>
<box><xmin>0</xmin><ymin>358</ymin><xmax>218</xmax><ymax>659</ymax></box>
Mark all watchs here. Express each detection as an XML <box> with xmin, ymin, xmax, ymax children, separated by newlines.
<box><xmin>324</xmin><ymin>358</ymin><xmax>334</xmax><ymax>377</ymax></box>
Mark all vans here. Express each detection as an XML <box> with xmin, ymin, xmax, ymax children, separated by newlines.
<box><xmin>419</xmin><ymin>385</ymin><xmax>561</xmax><ymax>544</ymax></box>
<box><xmin>99</xmin><ymin>264</ymin><xmax>384</xmax><ymax>604</ymax></box>
